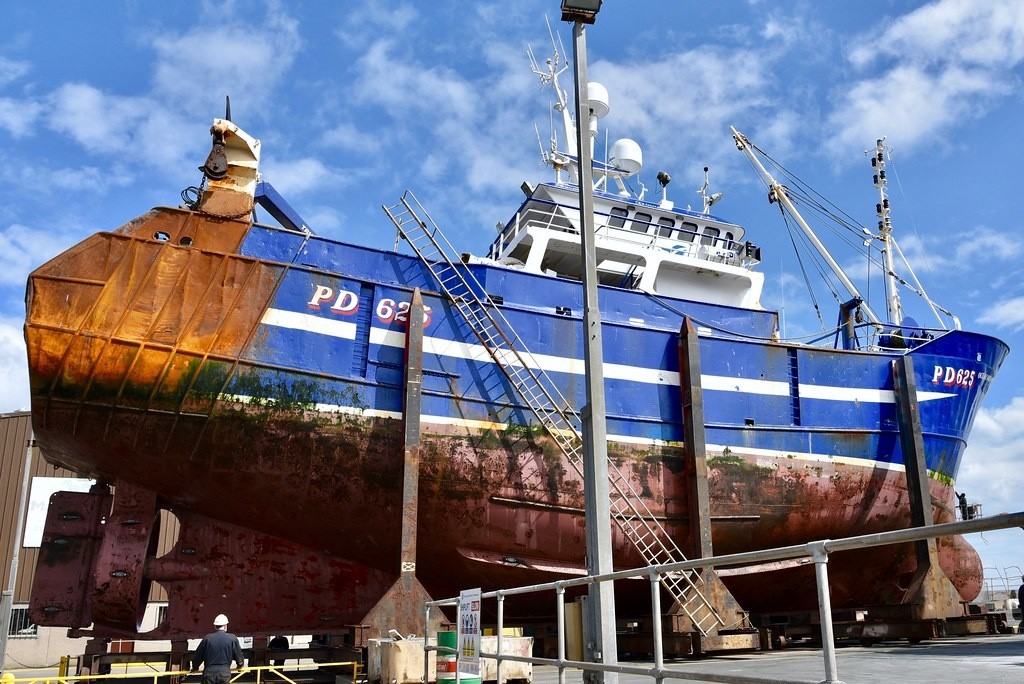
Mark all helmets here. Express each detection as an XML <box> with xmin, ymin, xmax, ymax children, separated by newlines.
<box><xmin>213</xmin><ymin>614</ymin><xmax>230</xmax><ymax>626</ymax></box>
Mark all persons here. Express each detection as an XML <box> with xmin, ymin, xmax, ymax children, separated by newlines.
<box><xmin>191</xmin><ymin>614</ymin><xmax>244</xmax><ymax>684</ymax></box>
<box><xmin>269</xmin><ymin>636</ymin><xmax>289</xmax><ymax>666</ymax></box>
<box><xmin>1018</xmin><ymin>575</ymin><xmax>1024</xmax><ymax>634</ymax></box>
<box><xmin>955</xmin><ymin>491</ymin><xmax>967</xmax><ymax>520</ymax></box>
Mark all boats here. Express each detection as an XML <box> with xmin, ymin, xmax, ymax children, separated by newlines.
<box><xmin>25</xmin><ymin>1</ymin><xmax>1011</xmax><ymax>656</ymax></box>
